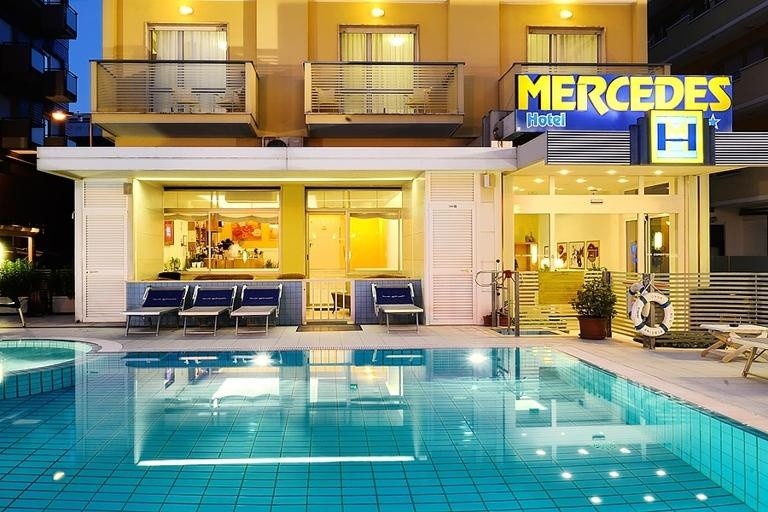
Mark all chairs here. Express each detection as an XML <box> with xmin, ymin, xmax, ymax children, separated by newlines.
<box><xmin>317</xmin><ymin>85</ymin><xmax>342</xmax><ymax>114</ymax></box>
<box><xmin>171</xmin><ymin>84</ymin><xmax>244</xmax><ymax>113</ymax></box>
<box><xmin>371</xmin><ymin>282</ymin><xmax>424</xmax><ymax>335</ymax></box>
<box><xmin>404</xmin><ymin>86</ymin><xmax>434</xmax><ymax>112</ymax></box>
<box><xmin>120</xmin><ymin>286</ymin><xmax>284</xmax><ymax>337</ymax></box>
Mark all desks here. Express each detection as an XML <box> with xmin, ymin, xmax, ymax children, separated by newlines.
<box><xmin>700</xmin><ymin>322</ymin><xmax>768</xmax><ymax>380</ymax></box>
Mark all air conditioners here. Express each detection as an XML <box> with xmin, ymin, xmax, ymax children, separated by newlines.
<box><xmin>262</xmin><ymin>136</ymin><xmax>304</xmax><ymax>149</ymax></box>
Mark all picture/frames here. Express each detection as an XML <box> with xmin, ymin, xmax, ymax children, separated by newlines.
<box><xmin>164</xmin><ymin>220</ymin><xmax>188</xmax><ymax>248</ymax></box>
<box><xmin>556</xmin><ymin>240</ymin><xmax>602</xmax><ymax>272</ymax></box>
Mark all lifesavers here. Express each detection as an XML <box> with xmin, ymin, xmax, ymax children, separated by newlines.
<box><xmin>627</xmin><ymin>283</ymin><xmax>650</xmax><ymax>323</ymax></box>
<box><xmin>631</xmin><ymin>292</ymin><xmax>673</xmax><ymax>335</ymax></box>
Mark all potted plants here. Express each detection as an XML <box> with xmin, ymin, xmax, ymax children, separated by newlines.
<box><xmin>572</xmin><ymin>279</ymin><xmax>617</xmax><ymax>340</ymax></box>
<box><xmin>190</xmin><ymin>251</ymin><xmax>208</xmax><ymax>270</ymax></box>
